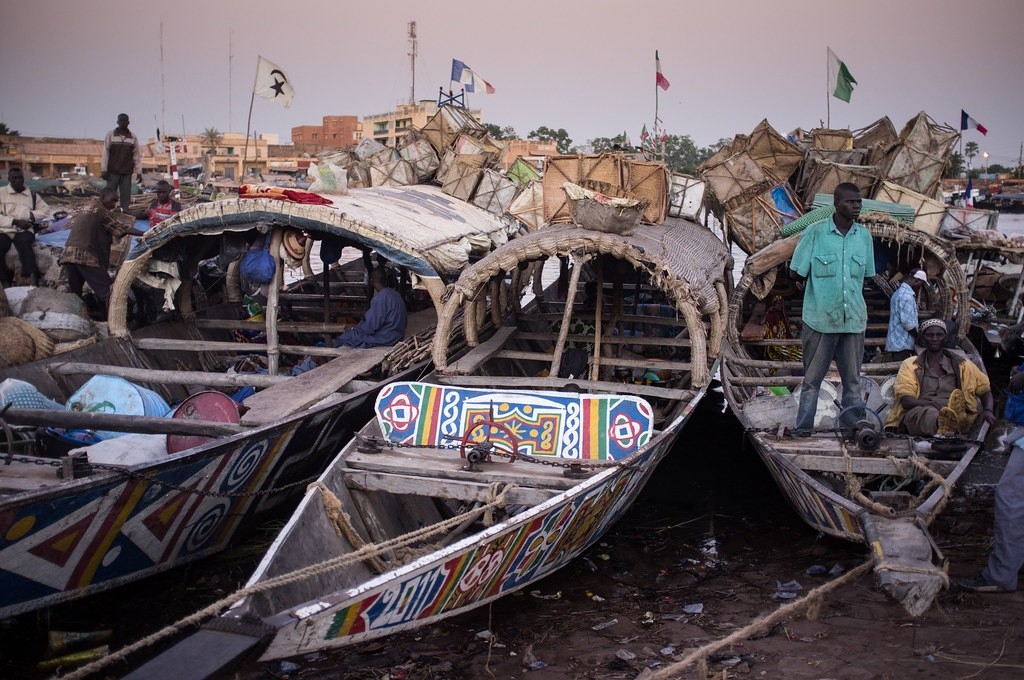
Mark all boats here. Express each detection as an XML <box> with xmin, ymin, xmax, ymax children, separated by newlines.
<box><xmin>718</xmin><ymin>199</ymin><xmax>994</xmax><ymax>616</ymax></box>
<box><xmin>937</xmin><ymin>207</ymin><xmax>1024</xmax><ymax>362</ymax></box>
<box><xmin>118</xmin><ymin>189</ymin><xmax>735</xmax><ymax>680</ymax></box>
<box><xmin>973</xmin><ymin>189</ymin><xmax>1024</xmax><ymax>213</ymax></box>
<box><xmin>0</xmin><ymin>185</ymin><xmax>506</xmax><ymax>680</ymax></box>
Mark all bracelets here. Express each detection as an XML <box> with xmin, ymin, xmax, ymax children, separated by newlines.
<box><xmin>120</xmin><ymin>207</ymin><xmax>123</xmax><ymax>213</ymax></box>
<box><xmin>985</xmin><ymin>409</ymin><xmax>993</xmax><ymax>413</ymax></box>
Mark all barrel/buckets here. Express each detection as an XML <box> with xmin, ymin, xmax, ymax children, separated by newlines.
<box><xmin>45</xmin><ymin>427</ymin><xmax>106</xmax><ymax>457</ymax></box>
<box><xmin>166</xmin><ymin>391</ymin><xmax>248</xmax><ymax>456</ymax></box>
<box><xmin>66</xmin><ymin>373</ymin><xmax>171</xmax><ymax>418</ymax></box>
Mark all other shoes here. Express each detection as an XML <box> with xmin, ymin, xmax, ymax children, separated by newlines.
<box><xmin>948</xmin><ymin>390</ymin><xmax>968</xmax><ymax>429</ymax></box>
<box><xmin>938</xmin><ymin>408</ymin><xmax>959</xmax><ymax>436</ymax></box>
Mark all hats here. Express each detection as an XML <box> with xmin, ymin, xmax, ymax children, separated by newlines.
<box><xmin>920</xmin><ymin>319</ymin><xmax>947</xmax><ymax>334</ymax></box>
<box><xmin>909</xmin><ymin>269</ymin><xmax>931</xmax><ymax>287</ymax></box>
<box><xmin>284</xmin><ymin>230</ymin><xmax>308</xmax><ymax>259</ymax></box>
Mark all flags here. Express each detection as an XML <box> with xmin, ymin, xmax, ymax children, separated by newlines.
<box><xmin>640</xmin><ymin>125</ymin><xmax>667</xmax><ymax>143</ymax></box>
<box><xmin>252</xmin><ymin>56</ymin><xmax>295</xmax><ymax>109</ymax></box>
<box><xmin>451</xmin><ymin>59</ymin><xmax>495</xmax><ymax>94</ymax></box>
<box><xmin>655</xmin><ymin>51</ymin><xmax>670</xmax><ymax>91</ymax></box>
<box><xmin>827</xmin><ymin>47</ymin><xmax>857</xmax><ymax>103</ymax></box>
<box><xmin>961</xmin><ymin>110</ymin><xmax>987</xmax><ymax>136</ymax></box>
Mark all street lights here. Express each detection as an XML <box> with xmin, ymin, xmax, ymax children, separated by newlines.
<box><xmin>983</xmin><ymin>152</ymin><xmax>988</xmax><ymax>179</ymax></box>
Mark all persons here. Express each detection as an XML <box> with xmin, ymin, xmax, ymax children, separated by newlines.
<box><xmin>884</xmin><ymin>318</ymin><xmax>997</xmax><ymax>437</ymax></box>
<box><xmin>533</xmin><ymin>294</ymin><xmax>687</xmax><ymax>390</ymax></box>
<box><xmin>100</xmin><ymin>114</ymin><xmax>143</xmax><ymax>214</ymax></box>
<box><xmin>0</xmin><ymin>168</ymin><xmax>50</xmax><ymax>288</ymax></box>
<box><xmin>790</xmin><ymin>182</ymin><xmax>875</xmax><ymax>442</ymax></box>
<box><xmin>333</xmin><ymin>268</ymin><xmax>407</xmax><ymax>348</ymax></box>
<box><xmin>59</xmin><ymin>187</ymin><xmax>145</xmax><ymax>329</ymax></box>
<box><xmin>114</xmin><ymin>181</ymin><xmax>181</xmax><ymax>262</ymax></box>
<box><xmin>958</xmin><ymin>361</ymin><xmax>1024</xmax><ymax>592</ymax></box>
<box><xmin>885</xmin><ymin>269</ymin><xmax>931</xmax><ymax>362</ymax></box>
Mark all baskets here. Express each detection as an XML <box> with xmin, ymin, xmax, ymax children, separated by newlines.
<box><xmin>563</xmin><ymin>179</ymin><xmax>652</xmax><ymax>236</ymax></box>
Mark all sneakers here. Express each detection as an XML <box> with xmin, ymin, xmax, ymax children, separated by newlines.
<box><xmin>958</xmin><ymin>570</ymin><xmax>1016</xmax><ymax>591</ymax></box>
